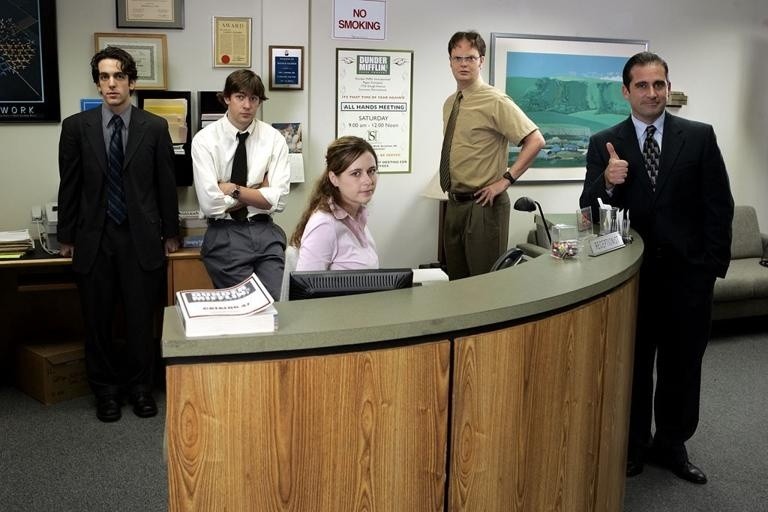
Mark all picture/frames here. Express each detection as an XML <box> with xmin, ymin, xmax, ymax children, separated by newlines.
<box><xmin>212</xmin><ymin>16</ymin><xmax>252</xmax><ymax>68</ymax></box>
<box><xmin>115</xmin><ymin>0</ymin><xmax>185</xmax><ymax>29</ymax></box>
<box><xmin>269</xmin><ymin>46</ymin><xmax>304</xmax><ymax>90</ymax></box>
<box><xmin>0</xmin><ymin>0</ymin><xmax>61</xmax><ymax>123</ymax></box>
<box><xmin>490</xmin><ymin>32</ymin><xmax>651</xmax><ymax>185</ymax></box>
<box><xmin>94</xmin><ymin>32</ymin><xmax>168</xmax><ymax>90</ymax></box>
<box><xmin>80</xmin><ymin>98</ymin><xmax>103</xmax><ymax>111</ymax></box>
<box><xmin>335</xmin><ymin>47</ymin><xmax>414</xmax><ymax>174</ymax></box>
<box><xmin>332</xmin><ymin>0</ymin><xmax>388</xmax><ymax>41</ymax></box>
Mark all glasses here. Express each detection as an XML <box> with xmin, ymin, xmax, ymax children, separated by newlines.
<box><xmin>451</xmin><ymin>56</ymin><xmax>482</xmax><ymax>65</ymax></box>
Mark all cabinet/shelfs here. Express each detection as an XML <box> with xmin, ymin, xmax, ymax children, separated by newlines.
<box><xmin>162</xmin><ymin>213</ymin><xmax>644</xmax><ymax>512</ymax></box>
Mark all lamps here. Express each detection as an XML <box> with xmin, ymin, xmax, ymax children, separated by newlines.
<box><xmin>514</xmin><ymin>197</ymin><xmax>551</xmax><ymax>248</ymax></box>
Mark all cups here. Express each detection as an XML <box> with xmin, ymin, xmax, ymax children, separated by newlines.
<box><xmin>600</xmin><ymin>207</ymin><xmax>619</xmax><ymax>235</ymax></box>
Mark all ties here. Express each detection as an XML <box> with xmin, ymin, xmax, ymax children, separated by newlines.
<box><xmin>104</xmin><ymin>117</ymin><xmax>128</xmax><ymax>228</ymax></box>
<box><xmin>231</xmin><ymin>132</ymin><xmax>250</xmax><ymax>223</ymax></box>
<box><xmin>440</xmin><ymin>91</ymin><xmax>462</xmax><ymax>193</ymax></box>
<box><xmin>642</xmin><ymin>126</ymin><xmax>663</xmax><ymax>192</ymax></box>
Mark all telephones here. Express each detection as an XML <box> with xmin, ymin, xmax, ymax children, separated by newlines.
<box><xmin>490</xmin><ymin>248</ymin><xmax>534</xmax><ymax>272</ymax></box>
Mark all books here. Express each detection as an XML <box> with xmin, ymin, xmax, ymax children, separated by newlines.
<box><xmin>0</xmin><ymin>229</ymin><xmax>35</xmax><ymax>260</ymax></box>
<box><xmin>175</xmin><ymin>273</ymin><xmax>278</xmax><ymax>339</ymax></box>
<box><xmin>180</xmin><ymin>210</ymin><xmax>207</xmax><ymax>247</ymax></box>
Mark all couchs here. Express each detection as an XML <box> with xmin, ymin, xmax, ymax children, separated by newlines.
<box><xmin>711</xmin><ymin>206</ymin><xmax>768</xmax><ymax>324</ymax></box>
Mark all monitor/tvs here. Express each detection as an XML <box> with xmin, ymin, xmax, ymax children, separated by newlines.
<box><xmin>285</xmin><ymin>269</ymin><xmax>415</xmax><ymax>302</ymax></box>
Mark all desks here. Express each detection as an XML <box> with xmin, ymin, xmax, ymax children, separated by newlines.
<box><xmin>0</xmin><ymin>240</ymin><xmax>214</xmax><ymax>306</ymax></box>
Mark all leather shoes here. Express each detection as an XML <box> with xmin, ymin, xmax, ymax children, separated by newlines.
<box><xmin>627</xmin><ymin>459</ymin><xmax>646</xmax><ymax>476</ymax></box>
<box><xmin>653</xmin><ymin>453</ymin><xmax>709</xmax><ymax>485</ymax></box>
<box><xmin>130</xmin><ymin>388</ymin><xmax>158</xmax><ymax>416</ymax></box>
<box><xmin>97</xmin><ymin>385</ymin><xmax>123</xmax><ymax>422</ymax></box>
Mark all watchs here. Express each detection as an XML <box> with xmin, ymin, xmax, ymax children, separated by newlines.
<box><xmin>230</xmin><ymin>183</ymin><xmax>241</xmax><ymax>201</ymax></box>
<box><xmin>504</xmin><ymin>172</ymin><xmax>516</xmax><ymax>181</ymax></box>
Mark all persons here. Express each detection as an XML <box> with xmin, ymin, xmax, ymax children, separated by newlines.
<box><xmin>58</xmin><ymin>47</ymin><xmax>180</xmax><ymax>423</ymax></box>
<box><xmin>579</xmin><ymin>52</ymin><xmax>735</xmax><ymax>484</ymax></box>
<box><xmin>294</xmin><ymin>136</ymin><xmax>379</xmax><ymax>272</ymax></box>
<box><xmin>440</xmin><ymin>32</ymin><xmax>546</xmax><ymax>281</ymax></box>
<box><xmin>192</xmin><ymin>70</ymin><xmax>290</xmax><ymax>302</ymax></box>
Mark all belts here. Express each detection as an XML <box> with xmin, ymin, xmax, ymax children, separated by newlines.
<box><xmin>448</xmin><ymin>189</ymin><xmax>480</xmax><ymax>202</ymax></box>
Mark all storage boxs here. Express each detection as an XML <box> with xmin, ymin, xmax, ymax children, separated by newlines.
<box><xmin>22</xmin><ymin>343</ymin><xmax>94</xmax><ymax>407</ymax></box>
